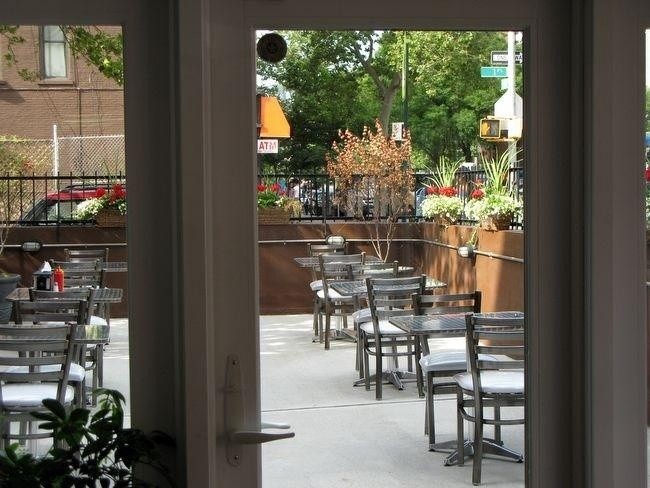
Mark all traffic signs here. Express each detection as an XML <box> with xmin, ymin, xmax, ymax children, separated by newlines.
<box><xmin>480</xmin><ymin>66</ymin><xmax>509</xmax><ymax>78</ymax></box>
<box><xmin>491</xmin><ymin>51</ymin><xmax>523</xmax><ymax>64</ymax></box>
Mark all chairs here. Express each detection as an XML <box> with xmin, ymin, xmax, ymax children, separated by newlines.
<box><xmin>0</xmin><ymin>247</ymin><xmax>128</xmax><ymax>458</ymax></box>
<box><xmin>410</xmin><ymin>291</ymin><xmax>483</xmax><ymax>436</ymax></box>
<box><xmin>294</xmin><ymin>244</ymin><xmax>447</xmax><ymax>397</ymax></box>
<box><xmin>463</xmin><ymin>311</ymin><xmax>525</xmax><ymax>485</ymax></box>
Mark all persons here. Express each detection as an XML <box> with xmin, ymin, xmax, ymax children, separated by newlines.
<box><xmin>292</xmin><ymin>179</ymin><xmax>303</xmax><ymax>201</ymax></box>
<box><xmin>285</xmin><ymin>182</ymin><xmax>294</xmax><ymax>200</ymax></box>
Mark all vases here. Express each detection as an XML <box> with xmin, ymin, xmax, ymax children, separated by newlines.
<box><xmin>258</xmin><ymin>207</ymin><xmax>290</xmax><ymax>225</ymax></box>
<box><xmin>436</xmin><ymin>218</ymin><xmax>454</xmax><ymax>225</ymax></box>
<box><xmin>96</xmin><ymin>209</ymin><xmax>125</xmax><ymax>227</ymax></box>
<box><xmin>490</xmin><ymin>215</ymin><xmax>512</xmax><ymax>231</ymax></box>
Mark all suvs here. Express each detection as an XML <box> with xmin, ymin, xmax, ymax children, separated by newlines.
<box><xmin>18</xmin><ymin>181</ymin><xmax>126</xmax><ymax>226</ymax></box>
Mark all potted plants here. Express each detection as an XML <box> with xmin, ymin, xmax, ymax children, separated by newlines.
<box><xmin>326</xmin><ymin>117</ymin><xmax>416</xmax><ymax>309</ymax></box>
<box><xmin>0</xmin><ymin>133</ymin><xmax>49</xmax><ymax>324</ymax></box>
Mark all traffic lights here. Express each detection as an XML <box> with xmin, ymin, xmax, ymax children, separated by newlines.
<box><xmin>478</xmin><ymin>117</ymin><xmax>504</xmax><ymax>141</ymax></box>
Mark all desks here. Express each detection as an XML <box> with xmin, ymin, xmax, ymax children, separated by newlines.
<box><xmin>389</xmin><ymin>311</ymin><xmax>525</xmax><ymax>467</ymax></box>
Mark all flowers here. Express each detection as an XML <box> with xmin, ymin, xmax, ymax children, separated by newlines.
<box><xmin>69</xmin><ymin>156</ymin><xmax>127</xmax><ymax>222</ymax></box>
<box><xmin>417</xmin><ymin>138</ymin><xmax>524</xmax><ymax>221</ymax></box>
<box><xmin>257</xmin><ymin>183</ymin><xmax>304</xmax><ymax>216</ymax></box>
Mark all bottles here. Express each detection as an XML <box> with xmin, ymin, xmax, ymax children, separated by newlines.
<box><xmin>55</xmin><ymin>264</ymin><xmax>64</xmax><ymax>291</ymax></box>
<box><xmin>53</xmin><ymin>282</ymin><xmax>59</xmax><ymax>291</ymax></box>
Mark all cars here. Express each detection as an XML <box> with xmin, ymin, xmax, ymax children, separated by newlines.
<box><xmin>296</xmin><ymin>186</ymin><xmax>466</xmax><ymax>221</ymax></box>
